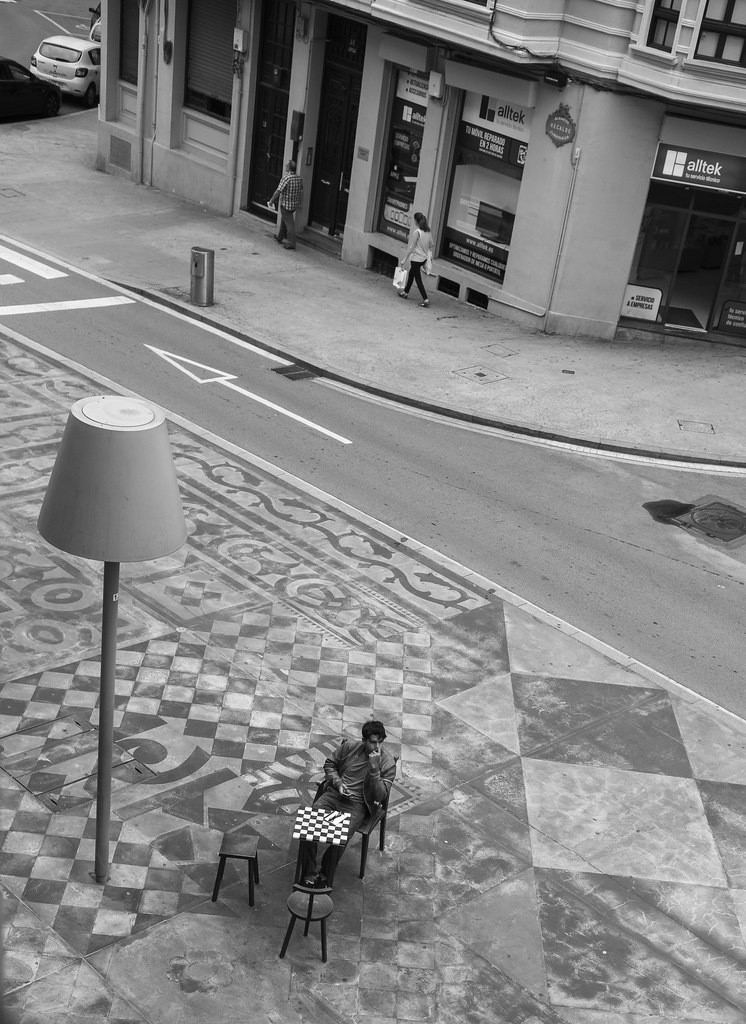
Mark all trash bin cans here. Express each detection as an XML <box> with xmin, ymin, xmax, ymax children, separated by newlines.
<box><xmin>190</xmin><ymin>246</ymin><xmax>215</xmax><ymax>307</ymax></box>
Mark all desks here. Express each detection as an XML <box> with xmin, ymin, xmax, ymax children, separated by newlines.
<box><xmin>293</xmin><ymin>806</ymin><xmax>351</xmax><ymax>893</ymax></box>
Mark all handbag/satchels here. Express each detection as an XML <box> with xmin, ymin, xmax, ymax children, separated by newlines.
<box><xmin>421</xmin><ymin>251</ymin><xmax>432</xmax><ymax>275</ymax></box>
<box><xmin>392</xmin><ymin>264</ymin><xmax>408</xmax><ymax>290</ymax></box>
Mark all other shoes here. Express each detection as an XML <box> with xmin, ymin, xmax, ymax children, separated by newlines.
<box><xmin>419</xmin><ymin>301</ymin><xmax>430</xmax><ymax>307</ymax></box>
<box><xmin>274</xmin><ymin>234</ymin><xmax>281</xmax><ymax>242</ymax></box>
<box><xmin>397</xmin><ymin>291</ymin><xmax>407</xmax><ymax>299</ymax></box>
<box><xmin>283</xmin><ymin>244</ymin><xmax>294</xmax><ymax>249</ymax></box>
<box><xmin>294</xmin><ymin>881</ymin><xmax>332</xmax><ymax>893</ymax></box>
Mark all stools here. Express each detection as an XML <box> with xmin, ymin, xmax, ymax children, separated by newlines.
<box><xmin>212</xmin><ymin>833</ymin><xmax>260</xmax><ymax>907</ymax></box>
<box><xmin>279</xmin><ymin>883</ymin><xmax>333</xmax><ymax>962</ymax></box>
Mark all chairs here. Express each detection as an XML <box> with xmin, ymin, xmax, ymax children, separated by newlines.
<box><xmin>313</xmin><ymin>738</ymin><xmax>399</xmax><ymax>878</ymax></box>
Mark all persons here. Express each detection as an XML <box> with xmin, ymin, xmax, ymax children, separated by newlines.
<box><xmin>300</xmin><ymin>721</ymin><xmax>395</xmax><ymax>888</ymax></box>
<box><xmin>268</xmin><ymin>160</ymin><xmax>303</xmax><ymax>249</ymax></box>
<box><xmin>397</xmin><ymin>212</ymin><xmax>435</xmax><ymax>307</ymax></box>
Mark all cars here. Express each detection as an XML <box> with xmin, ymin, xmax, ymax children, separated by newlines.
<box><xmin>0</xmin><ymin>56</ymin><xmax>62</xmax><ymax>124</ymax></box>
<box><xmin>88</xmin><ymin>3</ymin><xmax>101</xmax><ymax>45</ymax></box>
<box><xmin>30</xmin><ymin>33</ymin><xmax>100</xmax><ymax>107</ymax></box>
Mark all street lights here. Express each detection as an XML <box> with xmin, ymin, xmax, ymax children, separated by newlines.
<box><xmin>36</xmin><ymin>394</ymin><xmax>188</xmax><ymax>881</ymax></box>
